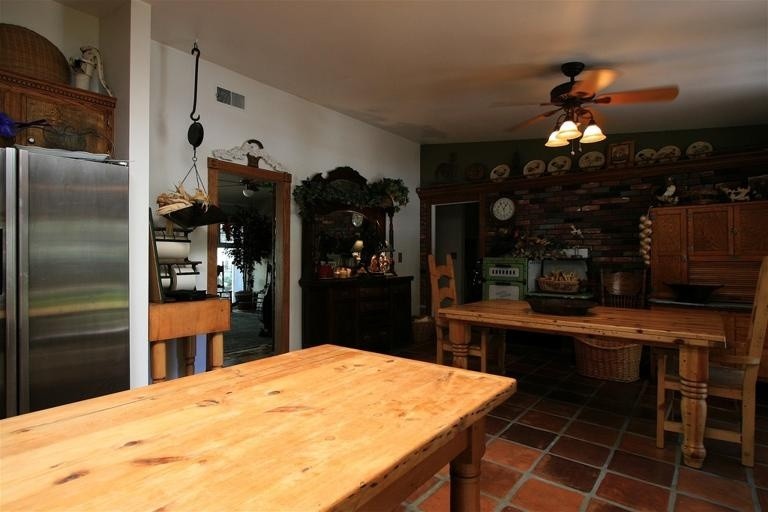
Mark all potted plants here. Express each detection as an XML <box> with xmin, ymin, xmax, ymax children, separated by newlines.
<box><xmin>222</xmin><ymin>202</ymin><xmax>273</xmax><ymax>313</ymax></box>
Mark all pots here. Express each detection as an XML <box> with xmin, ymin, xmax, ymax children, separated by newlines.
<box><xmin>660</xmin><ymin>280</ymin><xmax>726</xmax><ymax>302</ymax></box>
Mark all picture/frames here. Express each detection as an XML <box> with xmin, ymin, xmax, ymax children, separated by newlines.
<box><xmin>606</xmin><ymin>140</ymin><xmax>635</xmax><ymax>169</ymax></box>
<box><xmin>747</xmin><ymin>174</ymin><xmax>768</xmax><ymax>200</ymax></box>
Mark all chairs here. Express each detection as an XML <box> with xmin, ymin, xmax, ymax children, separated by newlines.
<box><xmin>428</xmin><ymin>253</ymin><xmax>507</xmax><ymax>375</ymax></box>
<box><xmin>655</xmin><ymin>253</ymin><xmax>767</xmax><ymax>467</ymax></box>
<box><xmin>597</xmin><ymin>267</ymin><xmax>648</xmax><ymax>379</ymax></box>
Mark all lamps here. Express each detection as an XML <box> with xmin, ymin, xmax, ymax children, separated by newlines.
<box><xmin>543</xmin><ymin>106</ymin><xmax>605</xmax><ymax>148</ymax></box>
<box><xmin>241</xmin><ymin>189</ymin><xmax>254</xmax><ymax>197</ymax></box>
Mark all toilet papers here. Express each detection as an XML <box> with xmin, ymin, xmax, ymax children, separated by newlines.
<box><xmin>157</xmin><ymin>241</ymin><xmax>191</xmax><ymax>260</ymax></box>
<box><xmin>171</xmin><ymin>267</ymin><xmax>196</xmax><ymax>293</ymax></box>
<box><xmin>160</xmin><ymin>271</ymin><xmax>171</xmax><ymax>288</ymax></box>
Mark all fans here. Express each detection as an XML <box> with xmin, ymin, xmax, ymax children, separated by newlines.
<box><xmin>486</xmin><ymin>58</ymin><xmax>679</xmax><ymax>136</ymax></box>
<box><xmin>217</xmin><ymin>175</ymin><xmax>272</xmax><ymax>192</ymax></box>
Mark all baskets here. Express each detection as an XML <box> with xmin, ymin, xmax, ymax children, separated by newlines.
<box><xmin>570</xmin><ymin>334</ymin><xmax>644</xmax><ymax>384</ymax></box>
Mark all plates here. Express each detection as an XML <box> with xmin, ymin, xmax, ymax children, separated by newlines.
<box><xmin>687</xmin><ymin>141</ymin><xmax>713</xmax><ymax>160</ymax></box>
<box><xmin>635</xmin><ymin>148</ymin><xmax>656</xmax><ymax>166</ymax></box>
<box><xmin>489</xmin><ymin>164</ymin><xmax>510</xmax><ymax>183</ymax></box>
<box><xmin>523</xmin><ymin>159</ymin><xmax>547</xmax><ymax>179</ymax></box>
<box><xmin>651</xmin><ymin>145</ymin><xmax>680</xmax><ymax>164</ymax></box>
<box><xmin>547</xmin><ymin>156</ymin><xmax>572</xmax><ymax>177</ymax></box>
<box><xmin>578</xmin><ymin>151</ymin><xmax>605</xmax><ymax>173</ymax></box>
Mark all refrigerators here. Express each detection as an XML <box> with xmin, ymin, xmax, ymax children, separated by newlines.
<box><xmin>1</xmin><ymin>144</ymin><xmax>131</xmax><ymax>420</ymax></box>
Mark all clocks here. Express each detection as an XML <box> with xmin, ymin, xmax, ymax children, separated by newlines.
<box><xmin>488</xmin><ymin>193</ymin><xmax>519</xmax><ymax>224</ymax></box>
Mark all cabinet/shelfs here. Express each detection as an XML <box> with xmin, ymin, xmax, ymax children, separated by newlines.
<box><xmin>0</xmin><ymin>70</ymin><xmax>118</xmax><ymax>156</ymax></box>
<box><xmin>297</xmin><ymin>275</ymin><xmax>414</xmax><ymax>356</ymax></box>
<box><xmin>647</xmin><ymin>197</ymin><xmax>767</xmax><ymax>296</ymax></box>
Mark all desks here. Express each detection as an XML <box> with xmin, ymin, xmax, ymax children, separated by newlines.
<box><xmin>0</xmin><ymin>342</ymin><xmax>518</xmax><ymax>512</ymax></box>
<box><xmin>438</xmin><ymin>297</ymin><xmax>728</xmax><ymax>471</ymax></box>
<box><xmin>148</xmin><ymin>297</ymin><xmax>230</xmax><ymax>384</ymax></box>
